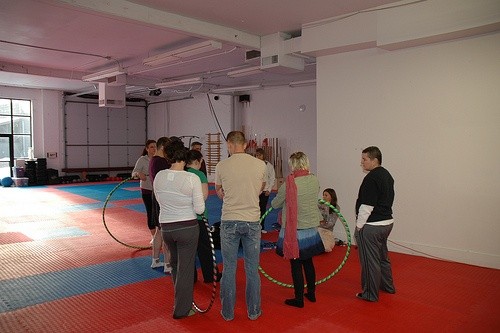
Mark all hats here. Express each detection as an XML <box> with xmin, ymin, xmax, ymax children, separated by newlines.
<box><xmin>190</xmin><ymin>138</ymin><xmax>203</xmax><ymax>145</ymax></box>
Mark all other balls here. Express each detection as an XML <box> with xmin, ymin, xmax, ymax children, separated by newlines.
<box><xmin>1</xmin><ymin>177</ymin><xmax>13</xmax><ymax>186</ymax></box>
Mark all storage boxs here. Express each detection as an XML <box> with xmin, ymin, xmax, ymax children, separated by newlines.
<box><xmin>11</xmin><ymin>158</ymin><xmax>59</xmax><ymax>187</ymax></box>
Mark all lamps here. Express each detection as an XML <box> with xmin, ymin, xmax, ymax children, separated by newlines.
<box><xmin>143</xmin><ymin>39</ymin><xmax>222</xmax><ymax>67</ymax></box>
<box><xmin>154</xmin><ymin>74</ymin><xmax>203</xmax><ymax>89</ymax></box>
<box><xmin>212</xmin><ymin>82</ymin><xmax>264</xmax><ymax>92</ymax></box>
<box><xmin>81</xmin><ymin>66</ymin><xmax>128</xmax><ymax>82</ymax></box>
<box><xmin>227</xmin><ymin>62</ymin><xmax>261</xmax><ymax>79</ymax></box>
<box><xmin>288</xmin><ymin>79</ymin><xmax>316</xmax><ymax>88</ymax></box>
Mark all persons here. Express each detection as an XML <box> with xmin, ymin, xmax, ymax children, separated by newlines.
<box><xmin>153</xmin><ymin>145</ymin><xmax>205</xmax><ymax>319</ymax></box>
<box><xmin>132</xmin><ymin>139</ymin><xmax>156</xmax><ymax>245</ymax></box>
<box><xmin>214</xmin><ymin>131</ymin><xmax>268</xmax><ymax>320</ymax></box>
<box><xmin>354</xmin><ymin>146</ymin><xmax>396</xmax><ymax>301</ymax></box>
<box><xmin>254</xmin><ymin>148</ymin><xmax>276</xmax><ymax>230</ymax></box>
<box><xmin>271</xmin><ymin>152</ymin><xmax>323</xmax><ymax>308</ymax></box>
<box><xmin>185</xmin><ymin>149</ymin><xmax>222</xmax><ymax>284</ymax></box>
<box><xmin>149</xmin><ymin>137</ymin><xmax>183</xmax><ymax>274</ymax></box>
<box><xmin>191</xmin><ymin>141</ymin><xmax>206</xmax><ymax>174</ymax></box>
<box><xmin>317</xmin><ymin>188</ymin><xmax>340</xmax><ymax>254</ymax></box>
